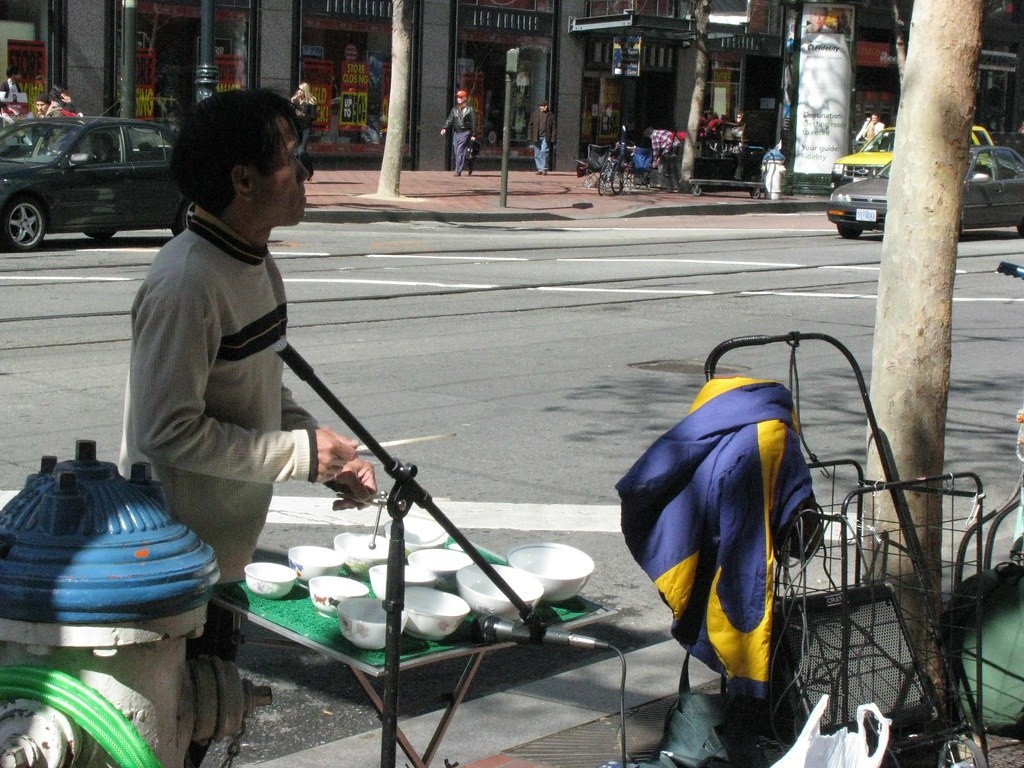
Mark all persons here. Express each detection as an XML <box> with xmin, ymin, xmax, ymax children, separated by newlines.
<box><xmin>528</xmin><ymin>102</ymin><xmax>556</xmax><ymax>175</ymax></box>
<box><xmin>116</xmin><ymin>88</ymin><xmax>377</xmax><ymax>768</ymax></box>
<box><xmin>440</xmin><ymin>90</ymin><xmax>476</xmax><ymax>177</ymax></box>
<box><xmin>606</xmin><ymin>102</ymin><xmax>746</xmax><ymax>186</ymax></box>
<box><xmin>87</xmin><ymin>132</ymin><xmax>114</xmax><ymax>165</ymax></box>
<box><xmin>809</xmin><ymin>7</ymin><xmax>849</xmax><ymax>34</ymax></box>
<box><xmin>291</xmin><ymin>83</ymin><xmax>317</xmax><ymax>182</ymax></box>
<box><xmin>0</xmin><ymin>66</ymin><xmax>81</xmax><ymax>154</ymax></box>
<box><xmin>861</xmin><ymin>114</ymin><xmax>886</xmax><ymax>149</ymax></box>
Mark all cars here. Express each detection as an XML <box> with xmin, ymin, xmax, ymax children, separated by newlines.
<box><xmin>0</xmin><ymin>116</ymin><xmax>187</xmax><ymax>252</ymax></box>
<box><xmin>826</xmin><ymin>145</ymin><xmax>1024</xmax><ymax>238</ymax></box>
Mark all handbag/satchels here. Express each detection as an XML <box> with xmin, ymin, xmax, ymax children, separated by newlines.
<box><xmin>467</xmin><ymin>141</ymin><xmax>481</xmax><ymax>158</ymax></box>
<box><xmin>770</xmin><ymin>693</ymin><xmax>892</xmax><ymax>768</ymax></box>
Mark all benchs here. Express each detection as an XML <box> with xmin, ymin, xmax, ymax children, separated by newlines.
<box><xmin>688</xmin><ymin>179</ymin><xmax>765</xmax><ymax>198</ymax></box>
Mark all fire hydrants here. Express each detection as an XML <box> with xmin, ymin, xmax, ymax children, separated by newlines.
<box><xmin>0</xmin><ymin>440</ymin><xmax>272</xmax><ymax>768</ymax></box>
<box><xmin>761</xmin><ymin>148</ymin><xmax>787</xmax><ymax>201</ymax></box>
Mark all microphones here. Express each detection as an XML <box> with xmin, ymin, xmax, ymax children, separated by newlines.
<box><xmin>471</xmin><ymin>615</ymin><xmax>612</xmax><ymax>650</ymax></box>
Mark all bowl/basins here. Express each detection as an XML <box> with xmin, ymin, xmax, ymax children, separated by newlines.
<box><xmin>404</xmin><ymin>548</ymin><xmax>475</xmax><ymax>592</ymax></box>
<box><xmin>309</xmin><ymin>575</ymin><xmax>370</xmax><ymax>618</ymax></box>
<box><xmin>397</xmin><ymin>585</ymin><xmax>470</xmax><ymax>641</ymax></box>
<box><xmin>369</xmin><ymin>563</ymin><xmax>437</xmax><ymax>602</ymax></box>
<box><xmin>334</xmin><ymin>533</ymin><xmax>392</xmax><ymax>581</ymax></box>
<box><xmin>455</xmin><ymin>563</ymin><xmax>544</xmax><ymax>624</ymax></box>
<box><xmin>288</xmin><ymin>544</ymin><xmax>343</xmax><ymax>584</ymax></box>
<box><xmin>506</xmin><ymin>542</ymin><xmax>595</xmax><ymax>605</ymax></box>
<box><xmin>384</xmin><ymin>515</ymin><xmax>448</xmax><ymax>561</ymax></box>
<box><xmin>245</xmin><ymin>562</ymin><xmax>297</xmax><ymax>600</ymax></box>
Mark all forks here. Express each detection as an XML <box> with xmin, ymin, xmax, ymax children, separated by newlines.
<box><xmin>337</xmin><ymin>598</ymin><xmax>408</xmax><ymax>651</ymax></box>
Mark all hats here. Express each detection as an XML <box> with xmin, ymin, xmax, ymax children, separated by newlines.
<box><xmin>36</xmin><ymin>94</ymin><xmax>51</xmax><ymax>105</ymax></box>
<box><xmin>538</xmin><ymin>102</ymin><xmax>548</xmax><ymax>107</ymax></box>
<box><xmin>456</xmin><ymin>91</ymin><xmax>467</xmax><ymax>97</ymax></box>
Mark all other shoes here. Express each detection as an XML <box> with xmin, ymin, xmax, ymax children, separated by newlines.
<box><xmin>468</xmin><ymin>165</ymin><xmax>473</xmax><ymax>176</ymax></box>
<box><xmin>453</xmin><ymin>173</ymin><xmax>461</xmax><ymax>176</ymax></box>
<box><xmin>536</xmin><ymin>170</ymin><xmax>547</xmax><ymax>175</ymax></box>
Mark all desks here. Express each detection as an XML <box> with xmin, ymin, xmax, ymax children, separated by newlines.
<box><xmin>197</xmin><ymin>551</ymin><xmax>620</xmax><ymax>767</ymax></box>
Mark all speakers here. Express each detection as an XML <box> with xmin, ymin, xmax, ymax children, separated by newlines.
<box><xmin>749</xmin><ymin>582</ymin><xmax>938</xmax><ymax>740</ymax></box>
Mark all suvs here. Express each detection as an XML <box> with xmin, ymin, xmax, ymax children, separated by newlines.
<box><xmin>830</xmin><ymin>125</ymin><xmax>994</xmax><ymax>194</ymax></box>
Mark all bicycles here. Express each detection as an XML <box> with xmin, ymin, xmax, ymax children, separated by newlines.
<box><xmin>596</xmin><ymin>145</ymin><xmax>651</xmax><ymax>196</ymax></box>
